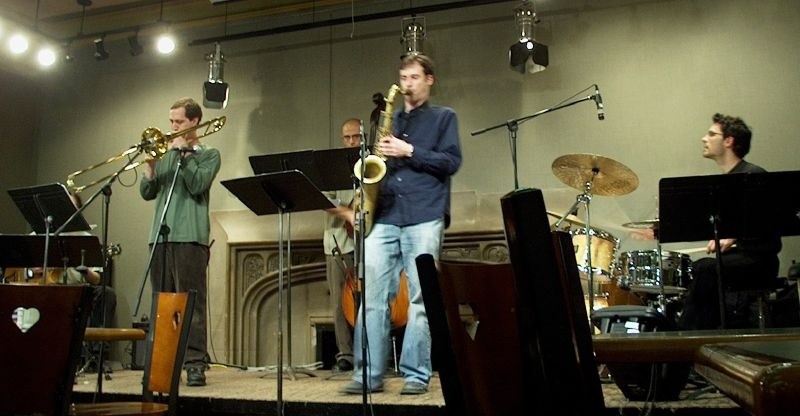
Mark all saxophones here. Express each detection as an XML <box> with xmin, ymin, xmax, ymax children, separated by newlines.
<box><xmin>353</xmin><ymin>85</ymin><xmax>411</xmax><ymax>239</ymax></box>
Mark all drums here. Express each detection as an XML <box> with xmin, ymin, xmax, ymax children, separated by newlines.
<box><xmin>567</xmin><ymin>229</ymin><xmax>621</xmax><ymax>285</ymax></box>
<box><xmin>622</xmin><ymin>249</ymin><xmax>694</xmax><ymax>296</ymax></box>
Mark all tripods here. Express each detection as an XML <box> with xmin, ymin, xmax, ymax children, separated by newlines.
<box><xmin>246</xmin><ymin>149</ymin><xmax>318</xmax><ymax>382</ymax></box>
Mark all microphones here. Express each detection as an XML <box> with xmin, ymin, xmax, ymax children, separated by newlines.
<box><xmin>594</xmin><ymin>84</ymin><xmax>604</xmax><ymax>120</ymax></box>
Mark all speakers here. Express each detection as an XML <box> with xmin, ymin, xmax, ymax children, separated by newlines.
<box><xmin>591</xmin><ymin>305</ymin><xmax>695</xmax><ymax>402</ymax></box>
<box><xmin>131</xmin><ymin>321</ymin><xmax>150</xmax><ymax>370</ymax></box>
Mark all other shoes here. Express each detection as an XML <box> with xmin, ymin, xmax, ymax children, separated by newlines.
<box><xmin>338</xmin><ymin>381</ymin><xmax>385</xmax><ymax>394</ymax></box>
<box><xmin>187</xmin><ymin>367</ymin><xmax>206</xmax><ymax>387</ymax></box>
<box><xmin>92</xmin><ymin>358</ymin><xmax>112</xmax><ymax>373</ymax></box>
<box><xmin>333</xmin><ymin>359</ymin><xmax>355</xmax><ymax>372</ymax></box>
<box><xmin>400</xmin><ymin>382</ymin><xmax>428</xmax><ymax>394</ymax></box>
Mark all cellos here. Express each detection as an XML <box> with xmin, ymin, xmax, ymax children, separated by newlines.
<box><xmin>341</xmin><ymin>93</ymin><xmax>411</xmax><ymax>375</ymax></box>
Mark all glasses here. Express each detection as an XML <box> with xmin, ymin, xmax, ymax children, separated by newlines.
<box><xmin>708</xmin><ymin>131</ymin><xmax>726</xmax><ymax>137</ymax></box>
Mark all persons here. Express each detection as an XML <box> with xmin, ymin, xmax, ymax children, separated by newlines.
<box><xmin>317</xmin><ymin>118</ymin><xmax>368</xmax><ymax>372</ymax></box>
<box><xmin>334</xmin><ymin>53</ymin><xmax>462</xmax><ymax>396</ymax></box>
<box><xmin>673</xmin><ymin>113</ymin><xmax>783</xmax><ymax>331</ymax></box>
<box><xmin>139</xmin><ymin>98</ymin><xmax>221</xmax><ymax>387</ymax></box>
<box><xmin>57</xmin><ymin>266</ymin><xmax>118</xmax><ymax>374</ymax></box>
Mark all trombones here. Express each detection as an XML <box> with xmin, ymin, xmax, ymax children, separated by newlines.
<box><xmin>67</xmin><ymin>114</ymin><xmax>228</xmax><ymax>194</ymax></box>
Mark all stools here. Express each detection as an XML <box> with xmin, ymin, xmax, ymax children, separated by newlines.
<box><xmin>735</xmin><ymin>276</ymin><xmax>789</xmax><ymax>329</ymax></box>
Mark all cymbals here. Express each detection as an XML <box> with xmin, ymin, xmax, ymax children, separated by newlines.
<box><xmin>551</xmin><ymin>153</ymin><xmax>640</xmax><ymax>197</ymax></box>
<box><xmin>545</xmin><ymin>210</ymin><xmax>595</xmax><ymax>229</ymax></box>
<box><xmin>623</xmin><ymin>219</ymin><xmax>662</xmax><ymax>229</ymax></box>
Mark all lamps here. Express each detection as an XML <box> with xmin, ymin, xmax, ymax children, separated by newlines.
<box><xmin>127</xmin><ymin>27</ymin><xmax>144</xmax><ymax>56</ymax></box>
<box><xmin>203</xmin><ymin>39</ymin><xmax>230</xmax><ymax>110</ymax></box>
<box><xmin>155</xmin><ymin>24</ymin><xmax>175</xmax><ymax>54</ymax></box>
<box><xmin>508</xmin><ymin>0</ymin><xmax>548</xmax><ymax>74</ymax></box>
<box><xmin>94</xmin><ymin>34</ymin><xmax>108</xmax><ymax>60</ymax></box>
<box><xmin>61</xmin><ymin>40</ymin><xmax>74</xmax><ymax>64</ymax></box>
<box><xmin>399</xmin><ymin>12</ymin><xmax>426</xmax><ymax>59</ymax></box>
